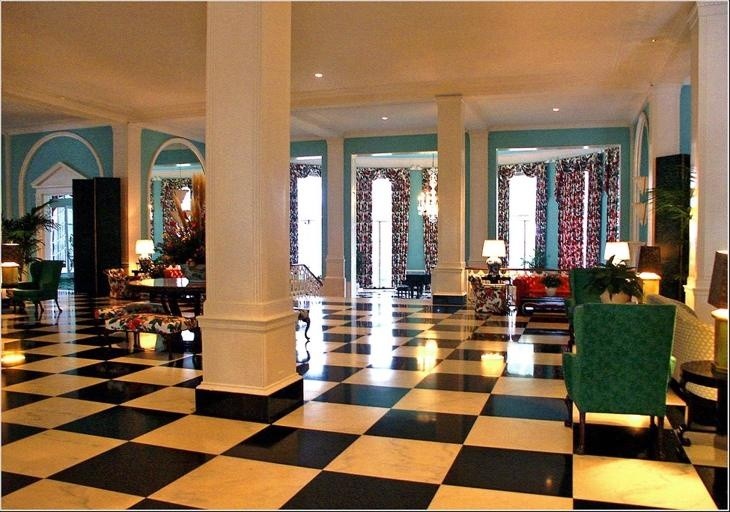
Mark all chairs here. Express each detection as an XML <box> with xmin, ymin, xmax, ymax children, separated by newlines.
<box><xmin>468</xmin><ymin>274</ymin><xmax>507</xmax><ymax>317</ymax></box>
<box><xmin>102</xmin><ymin>268</ymin><xmax>138</xmax><ymax>301</ymax></box>
<box><xmin>394</xmin><ymin>272</ymin><xmax>431</xmax><ymax>298</ymax></box>
<box><xmin>561</xmin><ymin>302</ymin><xmax>676</xmax><ymax>459</ymax></box>
<box><xmin>6</xmin><ymin>261</ymin><xmax>66</xmax><ymax>316</ymax></box>
<box><xmin>648</xmin><ymin>295</ymin><xmax>718</xmax><ymax>401</ymax></box>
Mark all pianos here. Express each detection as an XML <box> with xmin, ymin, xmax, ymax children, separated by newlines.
<box><xmin>403</xmin><ymin>275</ymin><xmax>431</xmax><ymax>297</ymax></box>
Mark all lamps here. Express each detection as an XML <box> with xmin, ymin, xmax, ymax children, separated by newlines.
<box><xmin>481</xmin><ymin>240</ymin><xmax>506</xmax><ymax>273</ymax></box>
<box><xmin>636</xmin><ymin>245</ymin><xmax>661</xmax><ymax>294</ymax></box>
<box><xmin>605</xmin><ymin>242</ymin><xmax>631</xmax><ymax>266</ymax></box>
<box><xmin>172</xmin><ymin>143</ymin><xmax>189</xmax><ymax>202</ymax></box>
<box><xmin>2</xmin><ymin>242</ymin><xmax>21</xmax><ymax>282</ymax></box>
<box><xmin>707</xmin><ymin>249</ymin><xmax>729</xmax><ymax>373</ymax></box>
<box><xmin>417</xmin><ymin>153</ymin><xmax>439</xmax><ymax>224</ymax></box>
<box><xmin>134</xmin><ymin>239</ymin><xmax>156</xmax><ymax>272</ymax></box>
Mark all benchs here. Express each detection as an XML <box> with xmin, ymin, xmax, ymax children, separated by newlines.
<box><xmin>105</xmin><ymin>312</ymin><xmax>199</xmax><ymax>355</ymax></box>
<box><xmin>95</xmin><ymin>301</ymin><xmax>160</xmax><ymax>347</ymax></box>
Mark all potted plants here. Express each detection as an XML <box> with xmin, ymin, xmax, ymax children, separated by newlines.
<box><xmin>583</xmin><ymin>257</ymin><xmax>643</xmax><ymax>304</ymax></box>
<box><xmin>541</xmin><ymin>274</ymin><xmax>560</xmax><ymax>296</ymax></box>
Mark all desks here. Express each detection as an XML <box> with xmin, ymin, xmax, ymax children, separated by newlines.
<box><xmin>680</xmin><ymin>360</ymin><xmax>728</xmax><ymax>446</ymax></box>
<box><xmin>128</xmin><ymin>278</ymin><xmax>205</xmax><ymax>319</ymax></box>
<box><xmin>520</xmin><ymin>297</ymin><xmax>567</xmax><ymax>316</ymax></box>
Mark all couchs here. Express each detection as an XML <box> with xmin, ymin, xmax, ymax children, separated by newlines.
<box><xmin>513</xmin><ymin>274</ymin><xmax>571</xmax><ymax>313</ymax></box>
